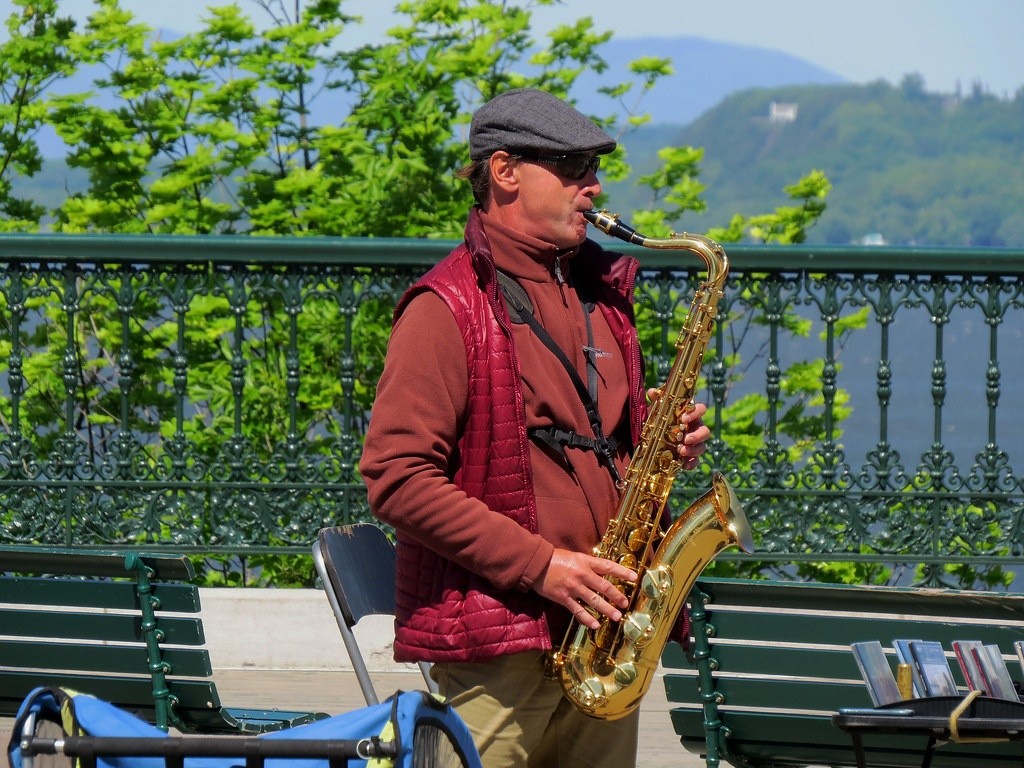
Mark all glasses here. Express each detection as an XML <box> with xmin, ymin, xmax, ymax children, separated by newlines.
<box><xmin>507</xmin><ymin>150</ymin><xmax>601</xmax><ymax>181</ymax></box>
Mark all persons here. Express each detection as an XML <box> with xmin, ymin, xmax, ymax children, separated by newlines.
<box><xmin>357</xmin><ymin>90</ymin><xmax>713</xmax><ymax>768</ymax></box>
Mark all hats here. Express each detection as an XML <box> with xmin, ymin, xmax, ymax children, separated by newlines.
<box><xmin>470</xmin><ymin>85</ymin><xmax>616</xmax><ymax>157</ymax></box>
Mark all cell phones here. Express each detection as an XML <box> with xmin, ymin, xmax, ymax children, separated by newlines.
<box><xmin>839</xmin><ymin>706</ymin><xmax>916</xmax><ymax>716</ymax></box>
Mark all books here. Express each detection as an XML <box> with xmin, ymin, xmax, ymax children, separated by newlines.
<box><xmin>893</xmin><ymin>638</ymin><xmax>1024</xmax><ymax>702</ymax></box>
<box><xmin>851</xmin><ymin>640</ymin><xmax>903</xmax><ymax>708</ymax></box>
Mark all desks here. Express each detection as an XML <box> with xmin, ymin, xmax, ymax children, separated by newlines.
<box><xmin>828</xmin><ymin>696</ymin><xmax>1024</xmax><ymax>768</ymax></box>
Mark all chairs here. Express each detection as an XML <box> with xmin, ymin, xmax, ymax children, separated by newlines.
<box><xmin>313</xmin><ymin>523</ymin><xmax>439</xmax><ymax>708</ymax></box>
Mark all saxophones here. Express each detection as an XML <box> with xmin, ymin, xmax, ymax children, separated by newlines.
<box><xmin>552</xmin><ymin>210</ymin><xmax>756</xmax><ymax>723</ymax></box>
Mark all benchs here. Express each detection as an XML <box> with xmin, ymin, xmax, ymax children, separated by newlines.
<box><xmin>1</xmin><ymin>542</ymin><xmax>333</xmax><ymax>737</ymax></box>
<box><xmin>660</xmin><ymin>578</ymin><xmax>1024</xmax><ymax>768</ymax></box>
